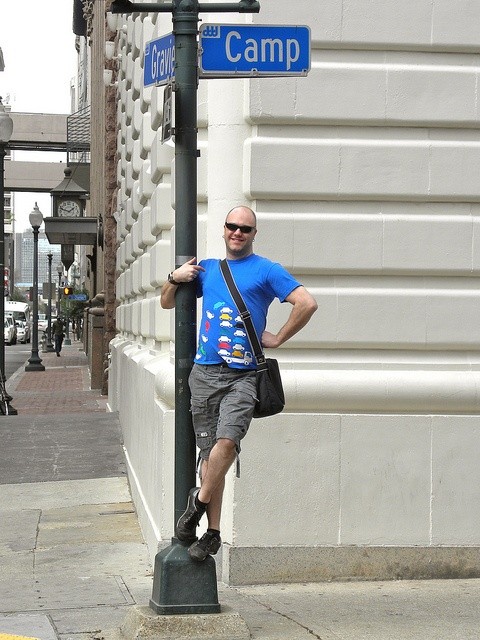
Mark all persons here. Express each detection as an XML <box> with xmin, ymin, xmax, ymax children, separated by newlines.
<box><xmin>51</xmin><ymin>317</ymin><xmax>67</xmax><ymax>358</ymax></box>
<box><xmin>160</xmin><ymin>206</ymin><xmax>317</xmax><ymax>560</ymax></box>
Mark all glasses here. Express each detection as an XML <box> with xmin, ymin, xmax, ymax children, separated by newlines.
<box><xmin>224</xmin><ymin>222</ymin><xmax>256</xmax><ymax>233</ymax></box>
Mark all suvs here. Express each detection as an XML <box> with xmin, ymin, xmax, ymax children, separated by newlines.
<box><xmin>16</xmin><ymin>320</ymin><xmax>28</xmax><ymax>344</ymax></box>
<box><xmin>4</xmin><ymin>315</ymin><xmax>17</xmax><ymax>346</ymax></box>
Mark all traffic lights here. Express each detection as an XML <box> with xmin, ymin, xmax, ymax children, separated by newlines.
<box><xmin>63</xmin><ymin>287</ymin><xmax>73</xmax><ymax>296</ymax></box>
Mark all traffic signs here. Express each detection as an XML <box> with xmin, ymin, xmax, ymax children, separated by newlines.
<box><xmin>142</xmin><ymin>30</ymin><xmax>178</xmax><ymax>87</ymax></box>
<box><xmin>198</xmin><ymin>22</ymin><xmax>313</xmax><ymax>77</ymax></box>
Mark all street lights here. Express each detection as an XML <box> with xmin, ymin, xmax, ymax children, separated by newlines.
<box><xmin>45</xmin><ymin>248</ymin><xmax>55</xmax><ymax>350</ymax></box>
<box><xmin>1</xmin><ymin>97</ymin><xmax>19</xmax><ymax>418</ymax></box>
<box><xmin>25</xmin><ymin>200</ymin><xmax>46</xmax><ymax>373</ymax></box>
<box><xmin>57</xmin><ymin>270</ymin><xmax>63</xmax><ymax>309</ymax></box>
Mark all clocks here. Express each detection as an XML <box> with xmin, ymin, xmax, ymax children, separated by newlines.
<box><xmin>51</xmin><ymin>167</ymin><xmax>87</xmax><ymax>217</ymax></box>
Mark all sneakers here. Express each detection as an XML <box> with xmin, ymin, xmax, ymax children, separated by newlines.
<box><xmin>176</xmin><ymin>487</ymin><xmax>209</xmax><ymax>540</ymax></box>
<box><xmin>189</xmin><ymin>533</ymin><xmax>221</xmax><ymax>561</ymax></box>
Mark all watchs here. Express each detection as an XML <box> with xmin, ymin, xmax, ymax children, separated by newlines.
<box><xmin>168</xmin><ymin>272</ymin><xmax>180</xmax><ymax>285</ymax></box>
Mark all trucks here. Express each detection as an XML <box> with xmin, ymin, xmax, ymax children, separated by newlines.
<box><xmin>5</xmin><ymin>301</ymin><xmax>30</xmax><ymax>321</ymax></box>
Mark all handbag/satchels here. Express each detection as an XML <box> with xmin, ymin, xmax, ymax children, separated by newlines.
<box><xmin>220</xmin><ymin>259</ymin><xmax>285</xmax><ymax>418</ymax></box>
